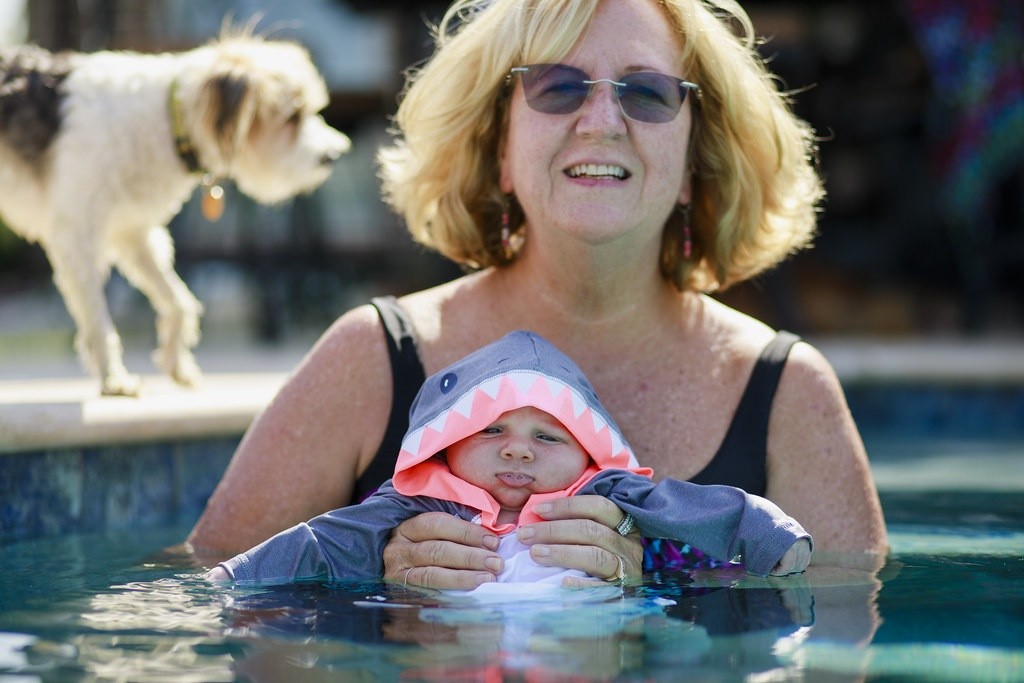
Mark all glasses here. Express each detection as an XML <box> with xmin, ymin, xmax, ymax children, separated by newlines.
<box><xmin>505</xmin><ymin>63</ymin><xmax>701</xmax><ymax>124</ymax></box>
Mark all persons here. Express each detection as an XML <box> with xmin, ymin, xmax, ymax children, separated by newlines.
<box><xmin>208</xmin><ymin>328</ymin><xmax>814</xmax><ymax>594</ymax></box>
<box><xmin>187</xmin><ymin>0</ymin><xmax>889</xmax><ymax>590</ymax></box>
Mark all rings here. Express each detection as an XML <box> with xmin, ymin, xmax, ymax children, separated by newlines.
<box><xmin>604</xmin><ymin>554</ymin><xmax>628</xmax><ymax>582</ymax></box>
<box><xmin>615</xmin><ymin>513</ymin><xmax>634</xmax><ymax>537</ymax></box>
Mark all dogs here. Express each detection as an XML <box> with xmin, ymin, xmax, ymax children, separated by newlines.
<box><xmin>0</xmin><ymin>8</ymin><xmax>352</xmax><ymax>397</ymax></box>
